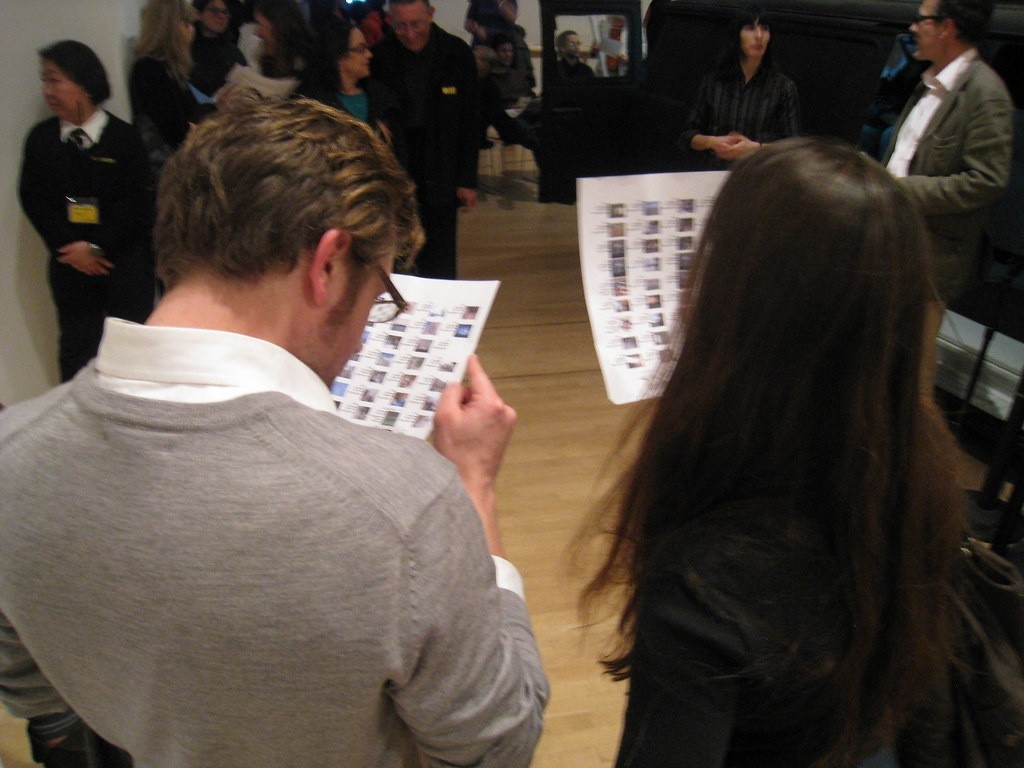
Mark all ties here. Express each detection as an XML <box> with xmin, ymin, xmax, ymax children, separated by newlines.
<box><xmin>67</xmin><ymin>128</ymin><xmax>85</xmax><ymax>151</ymax></box>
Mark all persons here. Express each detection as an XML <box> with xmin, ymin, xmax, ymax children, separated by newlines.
<box><xmin>590</xmin><ymin>139</ymin><xmax>1024</xmax><ymax>768</ymax></box>
<box><xmin>16</xmin><ymin>0</ymin><xmax>1024</xmax><ymax>387</ymax></box>
<box><xmin>0</xmin><ymin>95</ymin><xmax>551</xmax><ymax>768</ymax></box>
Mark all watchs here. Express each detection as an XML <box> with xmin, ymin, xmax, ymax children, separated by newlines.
<box><xmin>90</xmin><ymin>244</ymin><xmax>104</xmax><ymax>257</ymax></box>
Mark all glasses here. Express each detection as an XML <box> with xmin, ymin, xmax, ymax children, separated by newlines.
<box><xmin>202</xmin><ymin>6</ymin><xmax>233</xmax><ymax>19</ymax></box>
<box><xmin>362</xmin><ymin>250</ymin><xmax>408</xmax><ymax>324</ymax></box>
<box><xmin>913</xmin><ymin>14</ymin><xmax>960</xmax><ymax>28</ymax></box>
<box><xmin>342</xmin><ymin>43</ymin><xmax>369</xmax><ymax>55</ymax></box>
<box><xmin>392</xmin><ymin>5</ymin><xmax>429</xmax><ymax>35</ymax></box>
